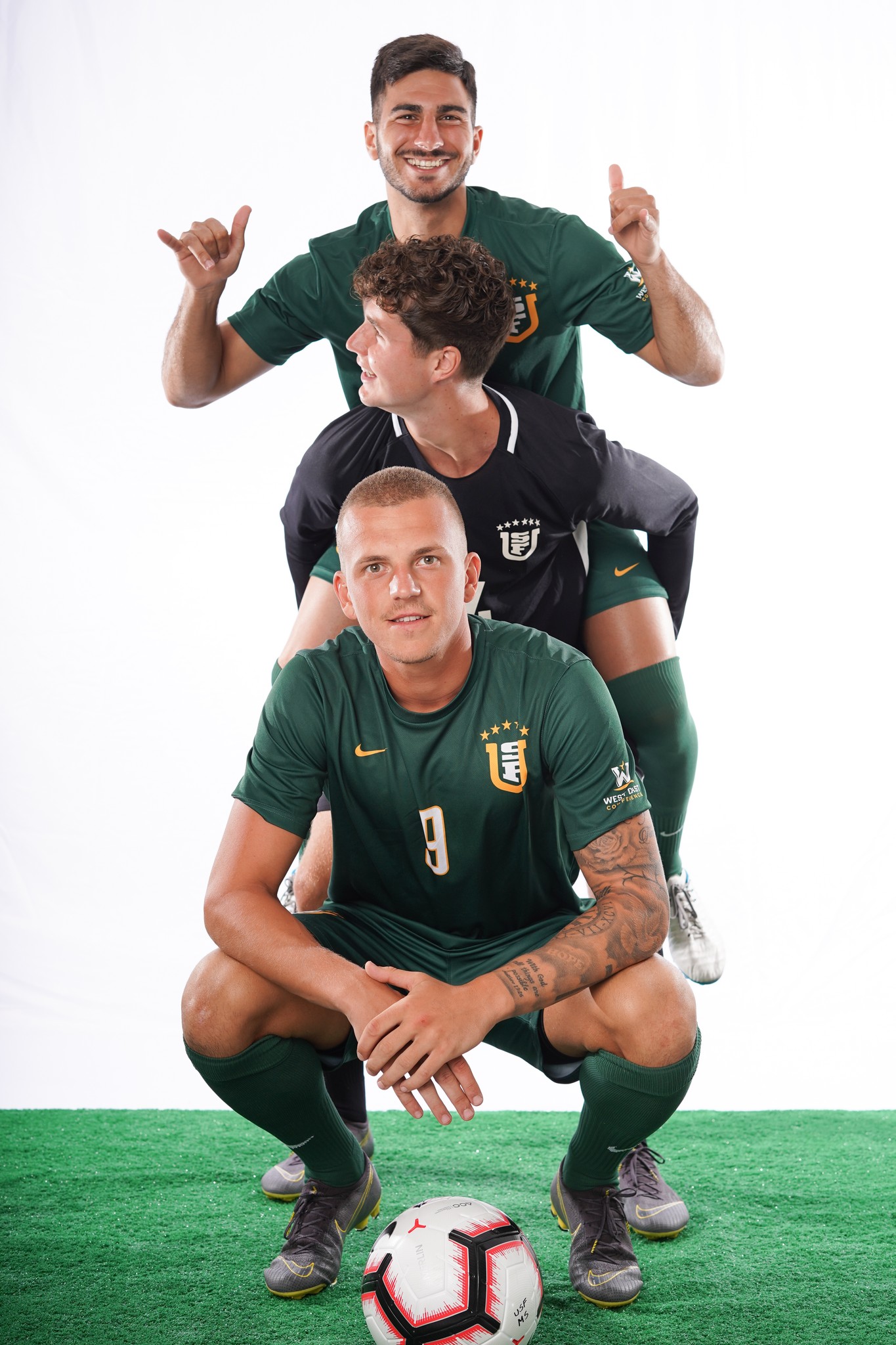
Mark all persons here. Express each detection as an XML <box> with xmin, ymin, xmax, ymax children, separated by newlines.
<box><xmin>181</xmin><ymin>465</ymin><xmax>698</xmax><ymax>1308</ymax></box>
<box><xmin>261</xmin><ymin>240</ymin><xmax>698</xmax><ymax>1240</ymax></box>
<box><xmin>158</xmin><ymin>31</ymin><xmax>725</xmax><ymax>990</ymax></box>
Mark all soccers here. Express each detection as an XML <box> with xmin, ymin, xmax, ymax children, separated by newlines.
<box><xmin>362</xmin><ymin>1197</ymin><xmax>544</xmax><ymax>1345</ymax></box>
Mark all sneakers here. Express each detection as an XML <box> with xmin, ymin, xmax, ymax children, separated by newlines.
<box><xmin>263</xmin><ymin>1147</ymin><xmax>382</xmax><ymax>1299</ymax></box>
<box><xmin>259</xmin><ymin>1118</ymin><xmax>375</xmax><ymax>1201</ymax></box>
<box><xmin>619</xmin><ymin>1144</ymin><xmax>689</xmax><ymax>1239</ymax></box>
<box><xmin>548</xmin><ymin>1154</ymin><xmax>644</xmax><ymax>1307</ymax></box>
<box><xmin>659</xmin><ymin>871</ymin><xmax>724</xmax><ymax>986</ymax></box>
